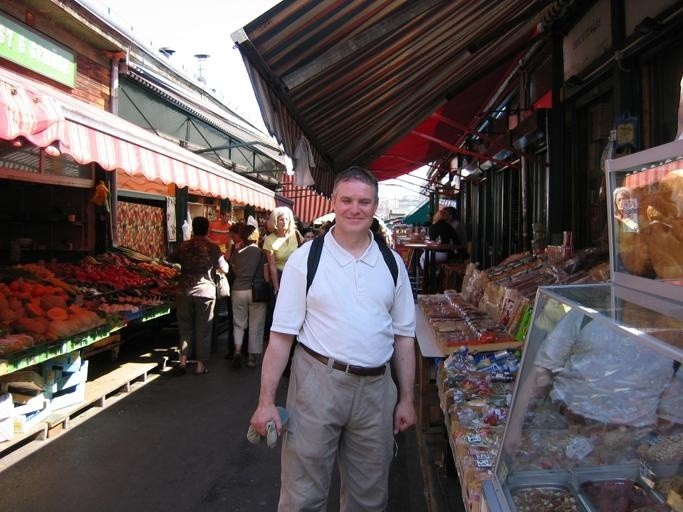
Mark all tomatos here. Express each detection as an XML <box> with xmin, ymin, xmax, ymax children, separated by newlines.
<box><xmin>88</xmin><ymin>268</ymin><xmax>151</xmax><ymax>289</ymax></box>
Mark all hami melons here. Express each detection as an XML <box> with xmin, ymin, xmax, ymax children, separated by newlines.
<box><xmin>0</xmin><ymin>282</ymin><xmax>101</xmax><ymax>341</ymax></box>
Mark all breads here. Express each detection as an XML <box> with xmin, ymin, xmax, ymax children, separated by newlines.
<box><xmin>620</xmin><ymin>170</ymin><xmax>683</xmax><ymax>279</ymax></box>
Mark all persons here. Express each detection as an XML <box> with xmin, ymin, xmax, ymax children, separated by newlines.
<box><xmin>246</xmin><ymin>163</ymin><xmax>419</xmax><ymax>512</ymax></box>
<box><xmin>612</xmin><ymin>186</ymin><xmax>632</xmax><ymax>223</ymax></box>
<box><xmin>418</xmin><ymin>204</ymin><xmax>470</xmax><ymax>282</ymax></box>
<box><xmin>153</xmin><ymin>210</ymin><xmax>337</xmax><ymax>379</ymax></box>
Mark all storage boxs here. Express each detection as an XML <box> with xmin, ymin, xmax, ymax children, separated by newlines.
<box><xmin>0</xmin><ymin>350</ymin><xmax>88</xmax><ymax>445</ymax></box>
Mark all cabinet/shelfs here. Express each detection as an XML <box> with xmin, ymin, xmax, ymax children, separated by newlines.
<box><xmin>476</xmin><ymin>139</ymin><xmax>683</xmax><ymax>511</ymax></box>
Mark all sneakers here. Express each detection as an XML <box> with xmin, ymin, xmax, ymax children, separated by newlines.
<box><xmin>225</xmin><ymin>352</ymin><xmax>261</xmax><ymax>369</ymax></box>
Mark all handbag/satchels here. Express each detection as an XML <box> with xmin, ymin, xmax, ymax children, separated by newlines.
<box><xmin>252</xmin><ymin>281</ymin><xmax>272</xmax><ymax>302</ymax></box>
<box><xmin>215</xmin><ymin>267</ymin><xmax>230</xmax><ymax>299</ymax></box>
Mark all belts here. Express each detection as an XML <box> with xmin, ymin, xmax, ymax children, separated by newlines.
<box><xmin>299</xmin><ymin>342</ymin><xmax>386</xmax><ymax>375</ymax></box>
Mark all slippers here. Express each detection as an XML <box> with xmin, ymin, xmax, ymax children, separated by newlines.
<box><xmin>176</xmin><ymin>365</ymin><xmax>186</xmax><ymax>373</ymax></box>
<box><xmin>195</xmin><ymin>367</ymin><xmax>208</xmax><ymax>375</ymax></box>
